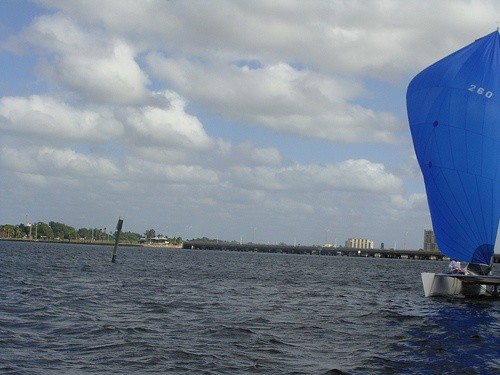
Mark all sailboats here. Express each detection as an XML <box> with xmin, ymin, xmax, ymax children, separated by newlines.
<box><xmin>406</xmin><ymin>27</ymin><xmax>500</xmax><ymax>298</ymax></box>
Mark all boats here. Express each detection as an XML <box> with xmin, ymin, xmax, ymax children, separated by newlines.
<box><xmin>142</xmin><ymin>236</ymin><xmax>184</xmax><ymax>248</ymax></box>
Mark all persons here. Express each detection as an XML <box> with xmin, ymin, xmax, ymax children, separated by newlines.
<box><xmin>447</xmin><ymin>257</ymin><xmax>473</xmax><ymax>275</ymax></box>
